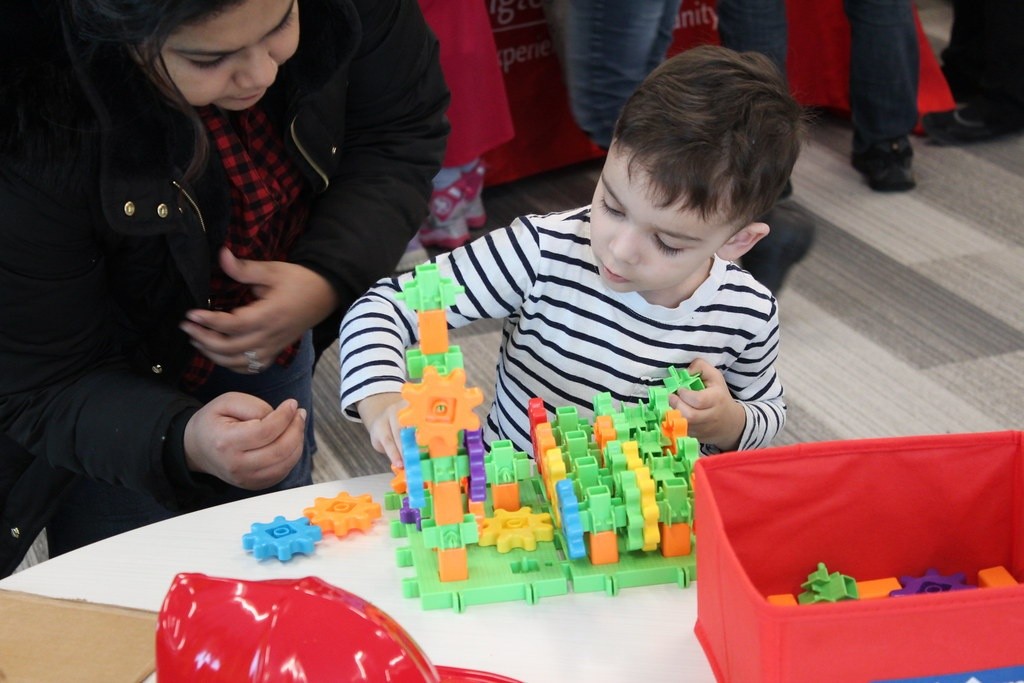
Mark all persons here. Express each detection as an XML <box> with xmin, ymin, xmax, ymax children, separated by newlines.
<box><xmin>924</xmin><ymin>0</ymin><xmax>1024</xmax><ymax>144</ymax></box>
<box><xmin>340</xmin><ymin>44</ymin><xmax>810</xmax><ymax>465</ymax></box>
<box><xmin>412</xmin><ymin>0</ymin><xmax>519</xmax><ymax>250</ymax></box>
<box><xmin>553</xmin><ymin>3</ymin><xmax>689</xmax><ymax>154</ymax></box>
<box><xmin>2</xmin><ymin>1</ymin><xmax>452</xmax><ymax>590</ymax></box>
<box><xmin>715</xmin><ymin>0</ymin><xmax>919</xmax><ymax>199</ymax></box>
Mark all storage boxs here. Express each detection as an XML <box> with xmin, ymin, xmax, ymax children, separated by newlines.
<box><xmin>694</xmin><ymin>432</ymin><xmax>1024</xmax><ymax>681</ymax></box>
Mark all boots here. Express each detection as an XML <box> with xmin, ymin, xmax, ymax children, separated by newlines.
<box><xmin>417</xmin><ymin>164</ymin><xmax>489</xmax><ymax>245</ymax></box>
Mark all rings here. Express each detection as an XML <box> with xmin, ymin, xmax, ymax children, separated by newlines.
<box><xmin>245</xmin><ymin>351</ymin><xmax>266</xmax><ymax>374</ymax></box>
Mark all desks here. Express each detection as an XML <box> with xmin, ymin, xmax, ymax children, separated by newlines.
<box><xmin>0</xmin><ymin>450</ymin><xmax>708</xmax><ymax>682</ymax></box>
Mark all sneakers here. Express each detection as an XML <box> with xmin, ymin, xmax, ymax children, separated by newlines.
<box><xmin>851</xmin><ymin>130</ymin><xmax>917</xmax><ymax>191</ymax></box>
<box><xmin>744</xmin><ymin>205</ymin><xmax>813</xmax><ymax>294</ymax></box>
<box><xmin>922</xmin><ymin>105</ymin><xmax>1009</xmax><ymax>141</ymax></box>
